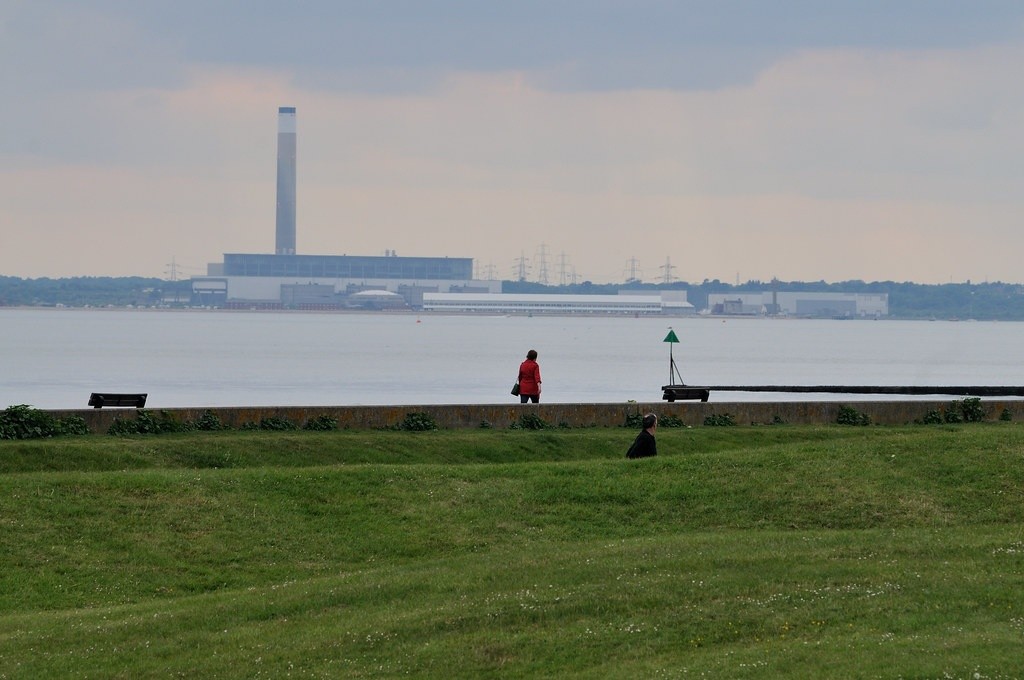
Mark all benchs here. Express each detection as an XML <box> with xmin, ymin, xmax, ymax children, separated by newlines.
<box><xmin>663</xmin><ymin>387</ymin><xmax>711</xmax><ymax>403</ymax></box>
<box><xmin>88</xmin><ymin>392</ymin><xmax>148</xmax><ymax>409</ymax></box>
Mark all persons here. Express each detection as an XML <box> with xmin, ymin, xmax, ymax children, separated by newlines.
<box><xmin>626</xmin><ymin>413</ymin><xmax>657</xmax><ymax>458</ymax></box>
<box><xmin>511</xmin><ymin>350</ymin><xmax>541</xmax><ymax>404</ymax></box>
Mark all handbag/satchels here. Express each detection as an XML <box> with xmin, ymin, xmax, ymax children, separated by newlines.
<box><xmin>511</xmin><ymin>383</ymin><xmax>520</xmax><ymax>396</ymax></box>
